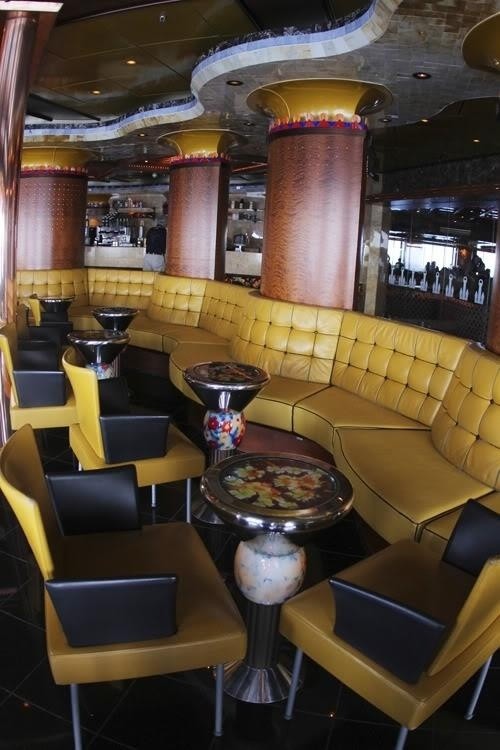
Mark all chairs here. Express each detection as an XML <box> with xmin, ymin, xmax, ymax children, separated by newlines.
<box><xmin>62</xmin><ymin>346</ymin><xmax>207</xmax><ymax>529</ymax></box>
<box><xmin>0</xmin><ymin>421</ymin><xmax>248</xmax><ymax>750</ymax></box>
<box><xmin>274</xmin><ymin>493</ymin><xmax>500</xmax><ymax>747</ymax></box>
<box><xmin>1</xmin><ymin>291</ymin><xmax>102</xmax><ymax>440</ymax></box>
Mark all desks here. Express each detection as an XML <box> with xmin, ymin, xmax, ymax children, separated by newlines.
<box><xmin>64</xmin><ymin>328</ymin><xmax>137</xmax><ymax>380</ymax></box>
<box><xmin>200</xmin><ymin>449</ymin><xmax>359</xmax><ymax>704</ymax></box>
<box><xmin>181</xmin><ymin>358</ymin><xmax>272</xmax><ymax>530</ymax></box>
<box><xmin>92</xmin><ymin>302</ymin><xmax>141</xmax><ymax>350</ymax></box>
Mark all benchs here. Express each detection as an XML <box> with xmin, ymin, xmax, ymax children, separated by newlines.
<box><xmin>236</xmin><ymin>290</ymin><xmax>470</xmax><ymax>454</ymax></box>
<box><xmin>17</xmin><ymin>266</ymin><xmax>158</xmax><ymax>337</ymax></box>
<box><xmin>127</xmin><ymin>271</ymin><xmax>254</xmax><ymax>405</ymax></box>
<box><xmin>333</xmin><ymin>338</ymin><xmax>500</xmax><ymax>571</ymax></box>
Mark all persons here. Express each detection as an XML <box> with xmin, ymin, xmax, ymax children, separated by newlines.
<box><xmin>142</xmin><ymin>214</ymin><xmax>167</xmax><ymax>272</ymax></box>
<box><xmin>387</xmin><ymin>255</ymin><xmax>486</xmax><ymax>271</ymax></box>
